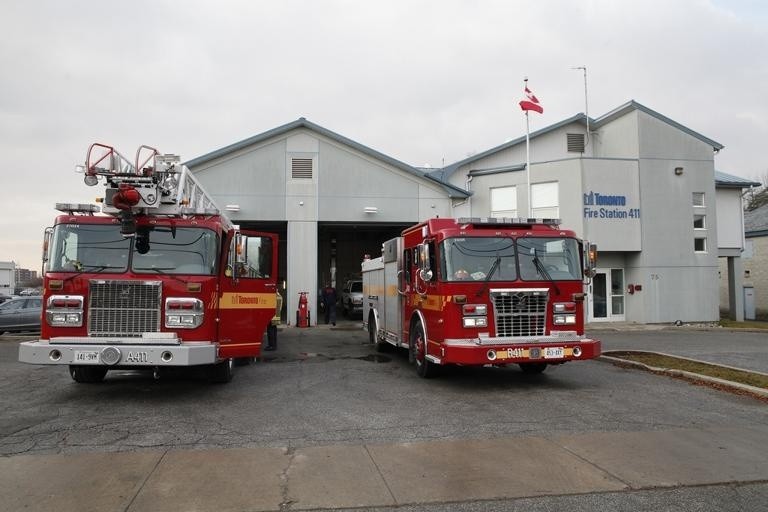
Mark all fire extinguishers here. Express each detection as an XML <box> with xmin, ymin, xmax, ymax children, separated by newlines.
<box><xmin>298</xmin><ymin>292</ymin><xmax>309</xmax><ymax>328</ymax></box>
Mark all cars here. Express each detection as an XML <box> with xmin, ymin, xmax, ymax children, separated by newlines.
<box><xmin>0</xmin><ymin>294</ymin><xmax>41</xmax><ymax>335</ymax></box>
<box><xmin>340</xmin><ymin>277</ymin><xmax>362</xmax><ymax>316</ymax></box>
<box><xmin>19</xmin><ymin>286</ymin><xmax>40</xmax><ymax>297</ymax></box>
<box><xmin>0</xmin><ymin>293</ymin><xmax>18</xmax><ymax>305</ymax></box>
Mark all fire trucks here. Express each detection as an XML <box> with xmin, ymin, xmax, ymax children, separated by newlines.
<box><xmin>16</xmin><ymin>141</ymin><xmax>284</xmax><ymax>389</ymax></box>
<box><xmin>358</xmin><ymin>210</ymin><xmax>601</xmax><ymax>380</ymax></box>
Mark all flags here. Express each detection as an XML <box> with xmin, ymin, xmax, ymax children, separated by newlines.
<box><xmin>519</xmin><ymin>87</ymin><xmax>543</xmax><ymax>113</ymax></box>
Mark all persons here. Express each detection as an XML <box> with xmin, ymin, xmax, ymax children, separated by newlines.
<box><xmin>263</xmin><ymin>289</ymin><xmax>284</xmax><ymax>350</ymax></box>
<box><xmin>320</xmin><ymin>282</ymin><xmax>338</xmax><ymax>326</ymax></box>
